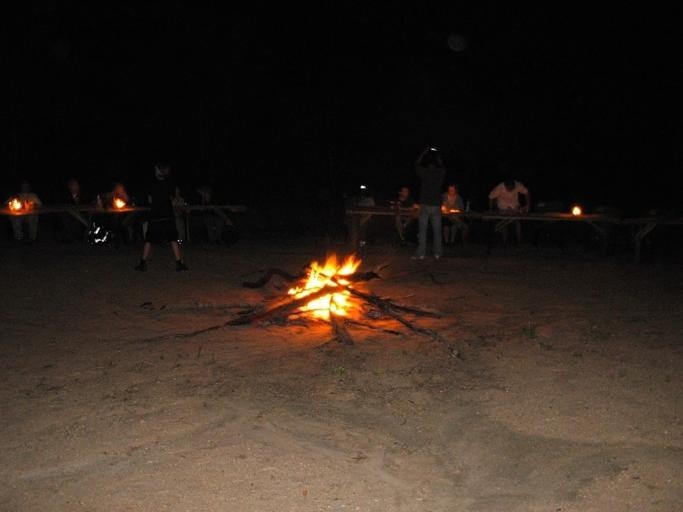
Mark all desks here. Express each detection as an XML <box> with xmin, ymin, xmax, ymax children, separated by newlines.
<box><xmin>478</xmin><ymin>208</ymin><xmax>657</xmax><ymax>253</ymax></box>
<box><xmin>345</xmin><ymin>203</ymin><xmax>471</xmax><ymax>260</ymax></box>
<box><xmin>2</xmin><ymin>201</ymin><xmax>244</xmax><ymax>248</ymax></box>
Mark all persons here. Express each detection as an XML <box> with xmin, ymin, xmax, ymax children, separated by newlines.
<box><xmin>413</xmin><ymin>143</ymin><xmax>445</xmax><ymax>259</ymax></box>
<box><xmin>4</xmin><ymin>178</ymin><xmax>236</xmax><ymax>252</ymax></box>
<box><xmin>136</xmin><ymin>158</ymin><xmax>190</xmax><ymax>271</ymax></box>
<box><xmin>351</xmin><ymin>174</ymin><xmax>529</xmax><ymax>257</ymax></box>
